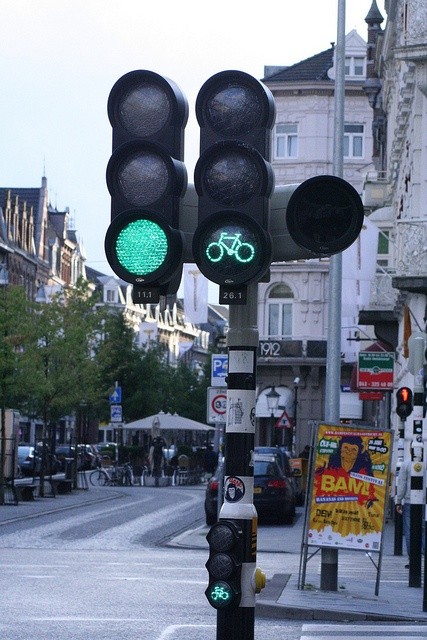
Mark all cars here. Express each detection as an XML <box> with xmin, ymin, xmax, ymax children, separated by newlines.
<box><xmin>43</xmin><ymin>441</ymin><xmax>92</xmax><ymax>475</ymax></box>
<box><xmin>16</xmin><ymin>446</ymin><xmax>43</xmax><ymax>476</ymax></box>
<box><xmin>254</xmin><ymin>446</ymin><xmax>286</xmax><ymax>474</ymax></box>
<box><xmin>93</xmin><ymin>442</ymin><xmax>117</xmax><ymax>460</ymax></box>
<box><xmin>204</xmin><ymin>455</ymin><xmax>296</xmax><ymax>526</ymax></box>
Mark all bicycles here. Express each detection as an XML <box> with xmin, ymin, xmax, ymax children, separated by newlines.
<box><xmin>89</xmin><ymin>460</ymin><xmax>133</xmax><ymax>487</ymax></box>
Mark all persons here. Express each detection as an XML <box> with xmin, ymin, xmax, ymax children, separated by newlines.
<box><xmin>393</xmin><ymin>457</ymin><xmax>426</xmax><ymax>569</ymax></box>
<box><xmin>171</xmin><ymin>445</ymin><xmax>217</xmax><ymax>483</ymax></box>
<box><xmin>149</xmin><ymin>434</ymin><xmax>167</xmax><ymax>477</ymax></box>
<box><xmin>298</xmin><ymin>445</ymin><xmax>310</xmax><ymax>459</ymax></box>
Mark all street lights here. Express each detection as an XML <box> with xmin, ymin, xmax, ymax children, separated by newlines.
<box><xmin>263</xmin><ymin>385</ymin><xmax>280</xmax><ymax>445</ymax></box>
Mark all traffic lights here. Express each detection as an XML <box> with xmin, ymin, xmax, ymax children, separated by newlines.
<box><xmin>106</xmin><ymin>69</ymin><xmax>188</xmax><ymax>286</ymax></box>
<box><xmin>192</xmin><ymin>69</ymin><xmax>277</xmax><ymax>287</ymax></box>
<box><xmin>205</xmin><ymin>520</ymin><xmax>243</xmax><ymax>610</ymax></box>
<box><xmin>284</xmin><ymin>174</ymin><xmax>364</xmax><ymax>258</ymax></box>
<box><xmin>395</xmin><ymin>387</ymin><xmax>412</xmax><ymax>418</ymax></box>
